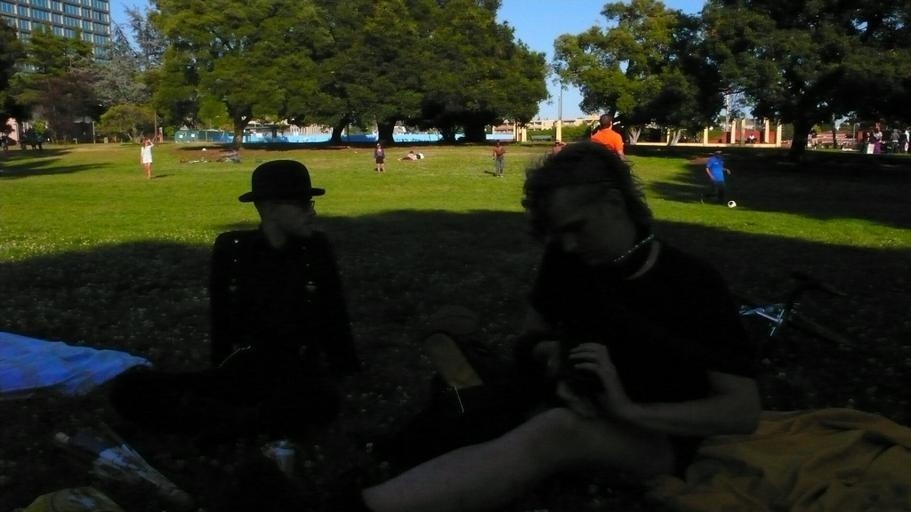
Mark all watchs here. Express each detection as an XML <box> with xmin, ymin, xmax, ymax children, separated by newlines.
<box><xmin>398</xmin><ymin>150</ymin><xmax>416</xmax><ymax>161</ymax></box>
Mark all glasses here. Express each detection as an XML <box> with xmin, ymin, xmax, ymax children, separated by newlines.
<box><xmin>290</xmin><ymin>201</ymin><xmax>316</xmax><ymax>211</ymax></box>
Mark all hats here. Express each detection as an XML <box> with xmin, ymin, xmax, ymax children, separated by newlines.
<box><xmin>240</xmin><ymin>159</ymin><xmax>324</xmax><ymax>204</ymax></box>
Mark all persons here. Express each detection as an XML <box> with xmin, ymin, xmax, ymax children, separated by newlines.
<box><xmin>138</xmin><ymin>137</ymin><xmax>154</xmax><ymax>181</ymax></box>
<box><xmin>860</xmin><ymin>117</ymin><xmax>910</xmax><ymax>157</ymax></box>
<box><xmin>491</xmin><ymin>138</ymin><xmax>506</xmax><ymax>177</ymax></box>
<box><xmin>697</xmin><ymin>148</ymin><xmax>731</xmax><ymax>205</ymax></box>
<box><xmin>811</xmin><ymin>135</ymin><xmax>818</xmax><ymax>151</ymax></box>
<box><xmin>590</xmin><ymin>113</ymin><xmax>625</xmax><ymax>161</ymax></box>
<box><xmin>107</xmin><ymin>161</ymin><xmax>363</xmax><ymax>456</ymax></box>
<box><xmin>371</xmin><ymin>139</ymin><xmax>385</xmax><ymax>173</ymax></box>
<box><xmin>552</xmin><ymin>142</ymin><xmax>567</xmax><ymax>155</ymax></box>
<box><xmin>313</xmin><ymin>141</ymin><xmax>760</xmax><ymax>512</ymax></box>
<box><xmin>807</xmin><ymin>130</ymin><xmax>818</xmax><ymax>151</ymax></box>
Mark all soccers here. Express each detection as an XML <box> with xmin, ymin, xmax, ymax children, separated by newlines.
<box><xmin>728</xmin><ymin>201</ymin><xmax>736</xmax><ymax>208</ymax></box>
